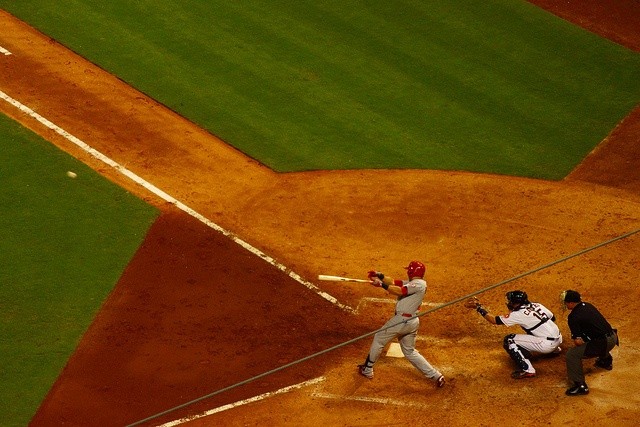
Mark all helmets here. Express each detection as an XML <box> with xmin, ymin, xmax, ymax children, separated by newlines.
<box><xmin>403</xmin><ymin>261</ymin><xmax>425</xmax><ymax>281</ymax></box>
<box><xmin>560</xmin><ymin>289</ymin><xmax>582</xmax><ymax>315</ymax></box>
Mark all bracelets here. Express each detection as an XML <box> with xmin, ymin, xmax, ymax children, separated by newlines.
<box><xmin>478</xmin><ymin>308</ymin><xmax>489</xmax><ymax>316</ymax></box>
<box><xmin>382</xmin><ymin>283</ymin><xmax>389</xmax><ymax>289</ymax></box>
<box><xmin>378</xmin><ymin>273</ymin><xmax>385</xmax><ymax>281</ymax></box>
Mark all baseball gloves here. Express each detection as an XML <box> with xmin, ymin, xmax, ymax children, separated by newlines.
<box><xmin>463</xmin><ymin>296</ymin><xmax>481</xmax><ymax>309</ymax></box>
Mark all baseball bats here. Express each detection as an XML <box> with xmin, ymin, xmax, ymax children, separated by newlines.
<box><xmin>318</xmin><ymin>274</ymin><xmax>375</xmax><ymax>284</ymax></box>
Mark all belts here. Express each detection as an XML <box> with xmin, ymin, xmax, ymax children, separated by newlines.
<box><xmin>395</xmin><ymin>312</ymin><xmax>412</xmax><ymax>317</ymax></box>
<box><xmin>605</xmin><ymin>331</ymin><xmax>615</xmax><ymax>337</ymax></box>
<box><xmin>546</xmin><ymin>337</ymin><xmax>555</xmax><ymax>341</ymax></box>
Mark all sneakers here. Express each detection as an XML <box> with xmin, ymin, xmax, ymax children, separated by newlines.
<box><xmin>505</xmin><ymin>291</ymin><xmax>528</xmax><ymax>310</ymax></box>
<box><xmin>359</xmin><ymin>363</ymin><xmax>374</xmax><ymax>379</ymax></box>
<box><xmin>437</xmin><ymin>376</ymin><xmax>445</xmax><ymax>387</ymax></box>
<box><xmin>511</xmin><ymin>371</ymin><xmax>536</xmax><ymax>378</ymax></box>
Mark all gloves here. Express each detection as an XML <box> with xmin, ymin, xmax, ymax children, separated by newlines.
<box><xmin>370</xmin><ymin>278</ymin><xmax>382</xmax><ymax>286</ymax></box>
<box><xmin>368</xmin><ymin>270</ymin><xmax>380</xmax><ymax>277</ymax></box>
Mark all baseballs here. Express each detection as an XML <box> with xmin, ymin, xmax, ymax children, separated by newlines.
<box><xmin>66</xmin><ymin>171</ymin><xmax>77</xmax><ymax>178</ymax></box>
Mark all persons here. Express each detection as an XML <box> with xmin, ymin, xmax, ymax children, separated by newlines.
<box><xmin>464</xmin><ymin>289</ymin><xmax>562</xmax><ymax>379</ymax></box>
<box><xmin>558</xmin><ymin>290</ymin><xmax>619</xmax><ymax>396</ymax></box>
<box><xmin>358</xmin><ymin>259</ymin><xmax>446</xmax><ymax>389</ymax></box>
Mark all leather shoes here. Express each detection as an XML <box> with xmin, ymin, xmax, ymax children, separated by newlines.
<box><xmin>566</xmin><ymin>386</ymin><xmax>589</xmax><ymax>394</ymax></box>
<box><xmin>595</xmin><ymin>359</ymin><xmax>613</xmax><ymax>369</ymax></box>
